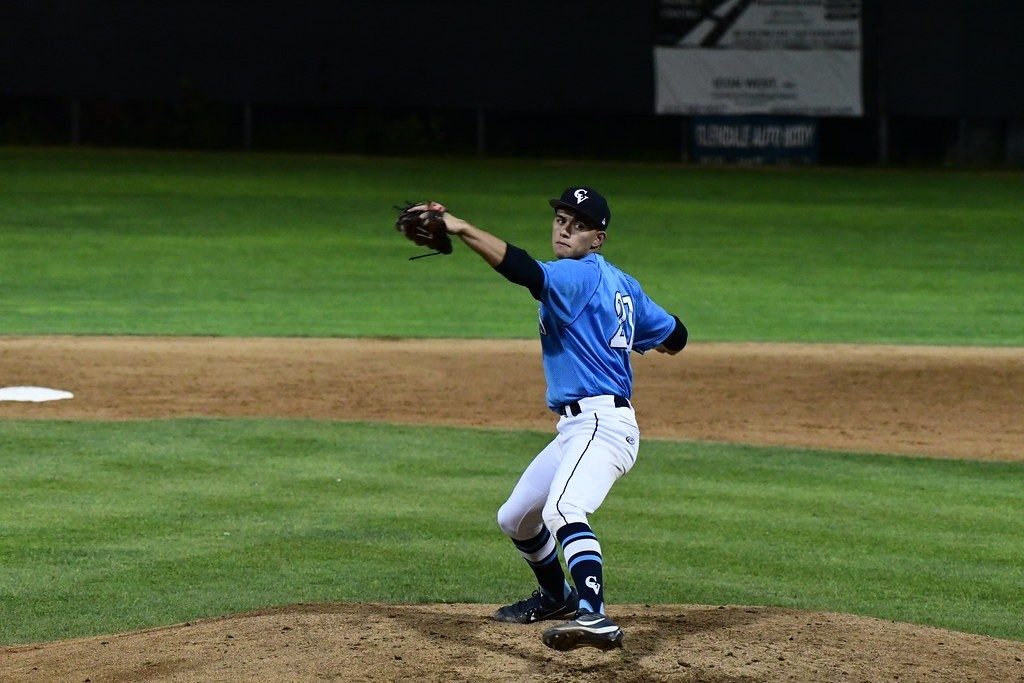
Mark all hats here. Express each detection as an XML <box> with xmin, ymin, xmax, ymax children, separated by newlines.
<box><xmin>549</xmin><ymin>186</ymin><xmax>610</xmax><ymax>231</ymax></box>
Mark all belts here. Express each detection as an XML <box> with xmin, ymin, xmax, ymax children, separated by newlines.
<box><xmin>560</xmin><ymin>394</ymin><xmax>630</xmax><ymax>418</ymax></box>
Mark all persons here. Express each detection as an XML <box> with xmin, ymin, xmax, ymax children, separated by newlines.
<box><xmin>406</xmin><ymin>185</ymin><xmax>688</xmax><ymax>652</ymax></box>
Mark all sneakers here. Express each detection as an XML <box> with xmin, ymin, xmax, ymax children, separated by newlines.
<box><xmin>544</xmin><ymin>614</ymin><xmax>623</xmax><ymax>652</ymax></box>
<box><xmin>496</xmin><ymin>584</ymin><xmax>579</xmax><ymax>622</ymax></box>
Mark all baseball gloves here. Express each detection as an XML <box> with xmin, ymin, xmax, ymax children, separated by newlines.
<box><xmin>393</xmin><ymin>201</ymin><xmax>455</xmax><ymax>257</ymax></box>
